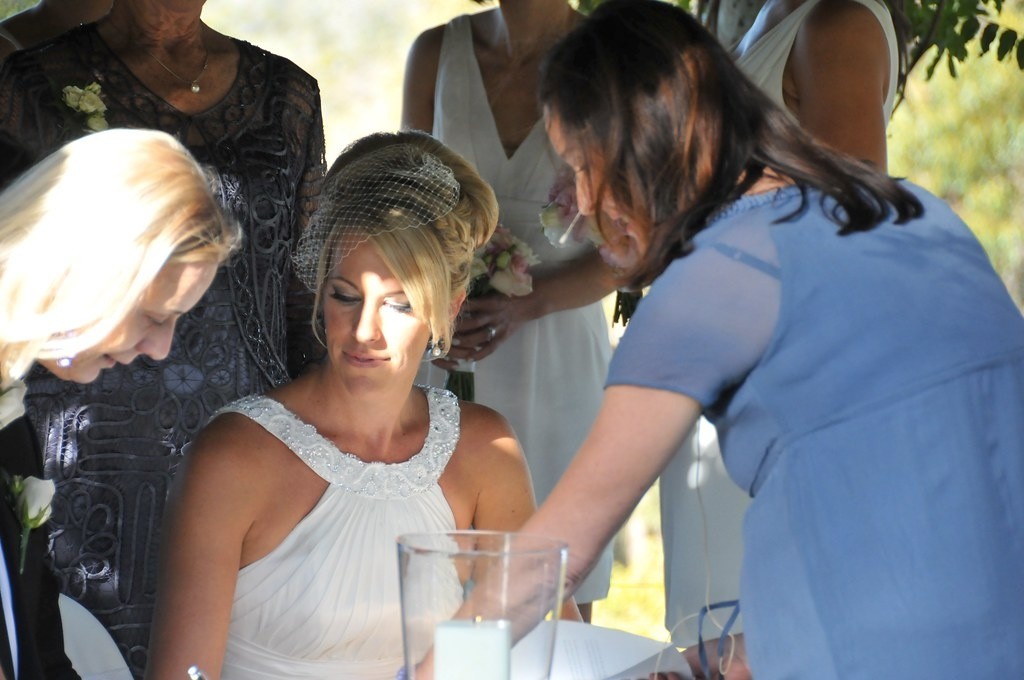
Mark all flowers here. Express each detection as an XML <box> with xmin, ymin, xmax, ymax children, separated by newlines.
<box><xmin>445</xmin><ymin>227</ymin><xmax>539</xmax><ymax>404</ymax></box>
<box><xmin>10</xmin><ymin>476</ymin><xmax>57</xmax><ymax>575</ymax></box>
<box><xmin>540</xmin><ymin>169</ymin><xmax>644</xmax><ymax>328</ymax></box>
<box><xmin>63</xmin><ymin>83</ymin><xmax>109</xmax><ymax>133</ymax></box>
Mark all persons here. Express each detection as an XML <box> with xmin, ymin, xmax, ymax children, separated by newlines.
<box><xmin>144</xmin><ymin>129</ymin><xmax>583</xmax><ymax>680</ymax></box>
<box><xmin>0</xmin><ymin>125</ymin><xmax>243</xmax><ymax>680</ymax></box>
<box><xmin>399</xmin><ymin>0</ymin><xmax>643</xmax><ymax>630</ymax></box>
<box><xmin>393</xmin><ymin>0</ymin><xmax>1024</xmax><ymax>680</ymax></box>
<box><xmin>657</xmin><ymin>0</ymin><xmax>898</xmax><ymax>680</ymax></box>
<box><xmin>0</xmin><ymin>0</ymin><xmax>327</xmax><ymax>680</ymax></box>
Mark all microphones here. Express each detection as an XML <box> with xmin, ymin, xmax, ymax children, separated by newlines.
<box><xmin>558</xmin><ymin>211</ymin><xmax>581</xmax><ymax>246</ymax></box>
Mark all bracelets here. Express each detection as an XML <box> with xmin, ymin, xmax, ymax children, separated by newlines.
<box><xmin>396</xmin><ymin>666</ymin><xmax>408</xmax><ymax>680</ymax></box>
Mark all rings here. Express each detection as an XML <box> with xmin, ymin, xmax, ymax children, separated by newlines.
<box><xmin>487</xmin><ymin>326</ymin><xmax>496</xmax><ymax>342</ymax></box>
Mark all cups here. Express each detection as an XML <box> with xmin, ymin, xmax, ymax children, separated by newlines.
<box><xmin>396</xmin><ymin>531</ymin><xmax>569</xmax><ymax>680</ymax></box>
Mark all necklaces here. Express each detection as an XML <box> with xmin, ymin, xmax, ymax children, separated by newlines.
<box><xmin>135</xmin><ymin>42</ymin><xmax>210</xmax><ymax>94</ymax></box>
